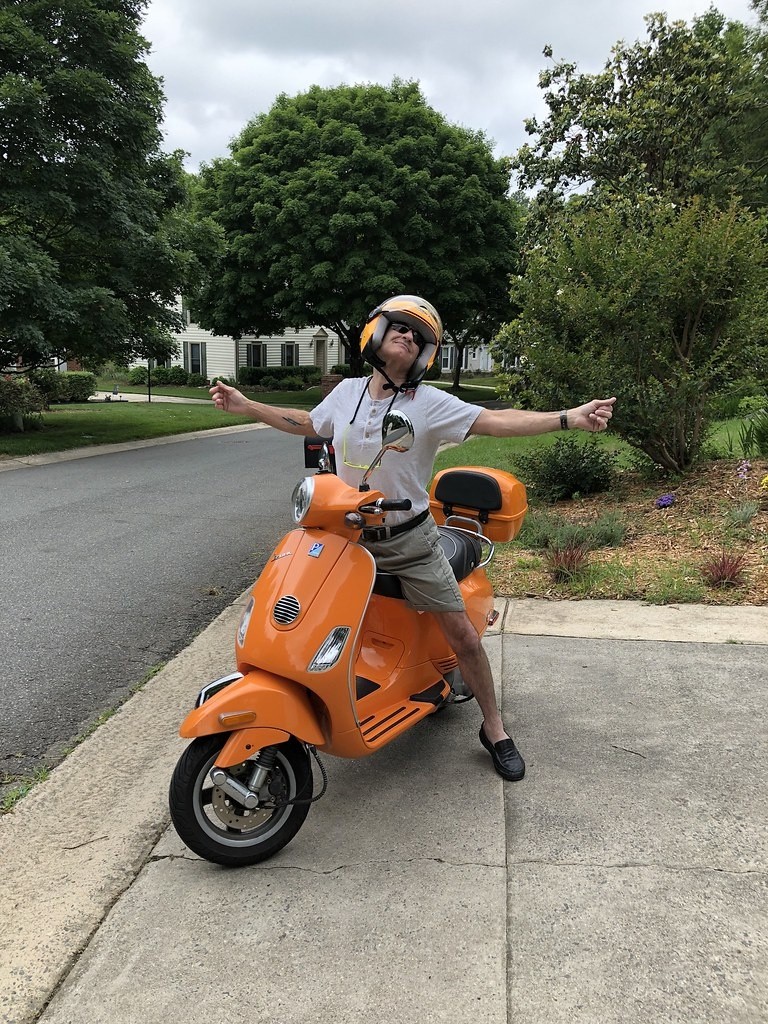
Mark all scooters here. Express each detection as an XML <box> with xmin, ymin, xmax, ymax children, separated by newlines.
<box><xmin>168</xmin><ymin>411</ymin><xmax>528</xmax><ymax>869</ymax></box>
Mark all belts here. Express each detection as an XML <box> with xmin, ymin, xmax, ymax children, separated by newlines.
<box><xmin>361</xmin><ymin>510</ymin><xmax>430</xmax><ymax>541</ymax></box>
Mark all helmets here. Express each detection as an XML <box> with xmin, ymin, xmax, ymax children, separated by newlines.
<box><xmin>360</xmin><ymin>295</ymin><xmax>442</xmax><ymax>383</ymax></box>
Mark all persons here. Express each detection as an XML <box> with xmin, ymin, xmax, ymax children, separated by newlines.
<box><xmin>209</xmin><ymin>295</ymin><xmax>617</xmax><ymax>781</ymax></box>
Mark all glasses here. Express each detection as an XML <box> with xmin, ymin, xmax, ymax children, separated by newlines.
<box><xmin>390</xmin><ymin>322</ymin><xmax>425</xmax><ymax>351</ymax></box>
<box><xmin>344</xmin><ymin>424</ymin><xmax>382</xmax><ymax>470</ymax></box>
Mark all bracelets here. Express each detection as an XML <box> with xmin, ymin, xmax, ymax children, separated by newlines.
<box><xmin>560</xmin><ymin>410</ymin><xmax>569</xmax><ymax>430</ymax></box>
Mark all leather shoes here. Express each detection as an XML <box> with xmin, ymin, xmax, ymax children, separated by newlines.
<box><xmin>479</xmin><ymin>721</ymin><xmax>525</xmax><ymax>780</ymax></box>
